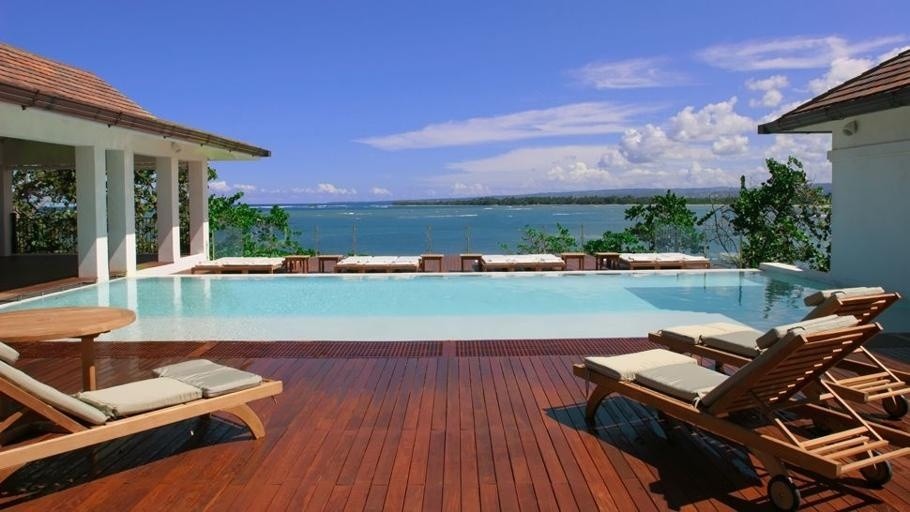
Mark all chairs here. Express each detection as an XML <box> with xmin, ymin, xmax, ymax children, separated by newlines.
<box><xmin>575</xmin><ymin>314</ymin><xmax>910</xmax><ymax>512</ymax></box>
<box><xmin>648</xmin><ymin>285</ymin><xmax>909</xmax><ymax>432</ymax></box>
<box><xmin>0</xmin><ymin>341</ymin><xmax>284</xmax><ymax>511</ymax></box>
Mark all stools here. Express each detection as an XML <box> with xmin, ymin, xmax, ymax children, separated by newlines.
<box><xmin>185</xmin><ymin>252</ymin><xmax>711</xmax><ymax>271</ymax></box>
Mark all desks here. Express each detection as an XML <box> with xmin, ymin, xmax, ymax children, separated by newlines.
<box><xmin>0</xmin><ymin>306</ymin><xmax>136</xmax><ymax>392</ymax></box>
